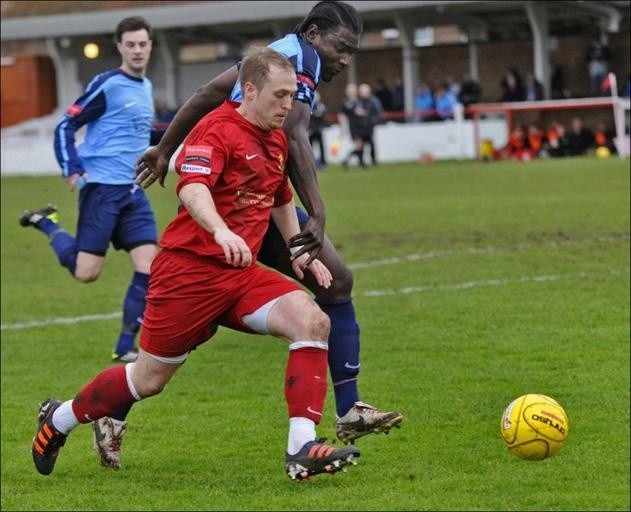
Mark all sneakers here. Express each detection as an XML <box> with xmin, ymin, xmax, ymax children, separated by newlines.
<box><xmin>29</xmin><ymin>398</ymin><xmax>69</xmax><ymax>476</ymax></box>
<box><xmin>282</xmin><ymin>439</ymin><xmax>362</xmax><ymax>483</ymax></box>
<box><xmin>17</xmin><ymin>204</ymin><xmax>60</xmax><ymax>229</ymax></box>
<box><xmin>332</xmin><ymin>400</ymin><xmax>403</xmax><ymax>445</ymax></box>
<box><xmin>90</xmin><ymin>414</ymin><xmax>131</xmax><ymax>469</ymax></box>
<box><xmin>109</xmin><ymin>344</ymin><xmax>139</xmax><ymax>362</ymax></box>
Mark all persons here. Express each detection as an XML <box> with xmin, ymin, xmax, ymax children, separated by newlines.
<box><xmin>131</xmin><ymin>0</ymin><xmax>403</xmax><ymax>448</ymax></box>
<box><xmin>17</xmin><ymin>13</ymin><xmax>161</xmax><ymax>364</ymax></box>
<box><xmin>308</xmin><ymin>91</ymin><xmax>328</xmax><ymax>167</ymax></box>
<box><xmin>338</xmin><ymin>33</ymin><xmax>624</xmax><ymax>168</ymax></box>
<box><xmin>31</xmin><ymin>53</ymin><xmax>359</xmax><ymax>486</ymax></box>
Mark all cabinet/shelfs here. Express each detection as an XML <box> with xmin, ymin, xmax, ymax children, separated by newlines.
<box><xmin>0</xmin><ymin>54</ymin><xmax>56</xmax><ymax>130</ymax></box>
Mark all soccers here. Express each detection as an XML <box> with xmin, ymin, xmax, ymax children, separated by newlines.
<box><xmin>500</xmin><ymin>393</ymin><xmax>567</xmax><ymax>461</ymax></box>
